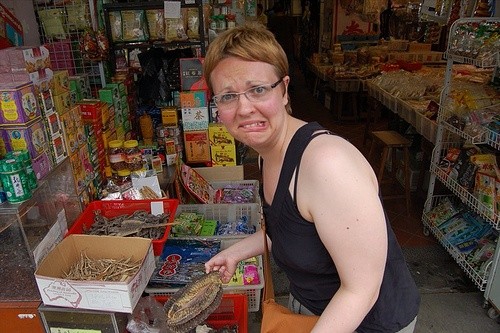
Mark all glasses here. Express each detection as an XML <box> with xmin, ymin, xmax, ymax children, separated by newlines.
<box><xmin>212</xmin><ymin>78</ymin><xmax>282</xmax><ymax>110</ymax></box>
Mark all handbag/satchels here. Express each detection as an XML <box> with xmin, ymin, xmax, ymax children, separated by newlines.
<box><xmin>261</xmin><ymin>298</ymin><xmax>320</xmax><ymax>333</ymax></box>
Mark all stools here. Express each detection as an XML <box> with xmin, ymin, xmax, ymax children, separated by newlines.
<box><xmin>366</xmin><ymin>130</ymin><xmax>412</xmax><ymax>216</ymax></box>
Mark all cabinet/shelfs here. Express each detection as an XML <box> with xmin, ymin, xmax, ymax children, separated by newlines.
<box><xmin>102</xmin><ymin>0</ymin><xmax>205</xmax><ymax>110</ymax></box>
<box><xmin>0</xmin><ymin>156</ymin><xmax>82</xmax><ymax>333</ymax></box>
<box><xmin>422</xmin><ymin>16</ymin><xmax>500</xmax><ymax>319</ymax></box>
<box><xmin>38</xmin><ymin>303</ymin><xmax>123</xmax><ymax>333</ymax></box>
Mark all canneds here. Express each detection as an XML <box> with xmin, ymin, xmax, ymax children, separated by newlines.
<box><xmin>108</xmin><ymin>140</ymin><xmax>143</xmax><ymax>172</ymax></box>
<box><xmin>210</xmin><ymin>15</ymin><xmax>236</xmax><ymax>30</ymax></box>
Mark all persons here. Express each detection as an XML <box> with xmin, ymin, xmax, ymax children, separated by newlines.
<box><xmin>124</xmin><ymin>48</ymin><xmax>173</xmax><ymax>135</ymax></box>
<box><xmin>203</xmin><ymin>21</ymin><xmax>420</xmax><ymax>333</ymax></box>
<box><xmin>268</xmin><ymin>0</ymin><xmax>298</xmax><ymax>96</ymax></box>
<box><xmin>255</xmin><ymin>2</ymin><xmax>274</xmax><ymax>27</ymax></box>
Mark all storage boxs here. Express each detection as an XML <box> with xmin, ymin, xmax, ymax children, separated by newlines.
<box><xmin>178</xmin><ymin>57</ymin><xmax>211</xmax><ymax>106</ymax></box>
<box><xmin>0</xmin><ymin>53</ymin><xmax>132</xmax><ymax>189</ymax></box>
<box><xmin>124</xmin><ymin>293</ymin><xmax>249</xmax><ymax>333</ymax></box>
<box><xmin>61</xmin><ymin>198</ymin><xmax>179</xmax><ymax>257</ymax></box>
<box><xmin>395</xmin><ymin>157</ymin><xmax>438</xmax><ymax>193</ymax></box>
<box><xmin>181</xmin><ymin>107</ymin><xmax>209</xmax><ymax>131</ymax></box>
<box><xmin>34</xmin><ymin>234</ymin><xmax>156</xmax><ymax>315</ymax></box>
<box><xmin>209</xmin><ymin>123</ymin><xmax>234</xmax><ymax>144</ymax></box>
<box><xmin>211</xmin><ymin>145</ymin><xmax>237</xmax><ymax>167</ymax></box>
<box><xmin>308</xmin><ymin>40</ymin><xmax>464</xmax><ymax>148</ymax></box>
<box><xmin>184</xmin><ymin>132</ymin><xmax>211</xmax><ymax>164</ymax></box>
<box><xmin>193</xmin><ymin>164</ymin><xmax>244</xmax><ymax>181</ymax></box>
<box><xmin>145</xmin><ymin>239</ymin><xmax>264</xmax><ymax>313</ymax></box>
<box><xmin>198</xmin><ymin>180</ymin><xmax>259</xmax><ymax>204</ymax></box>
<box><xmin>168</xmin><ymin>203</ymin><xmax>261</xmax><ymax>240</ymax></box>
